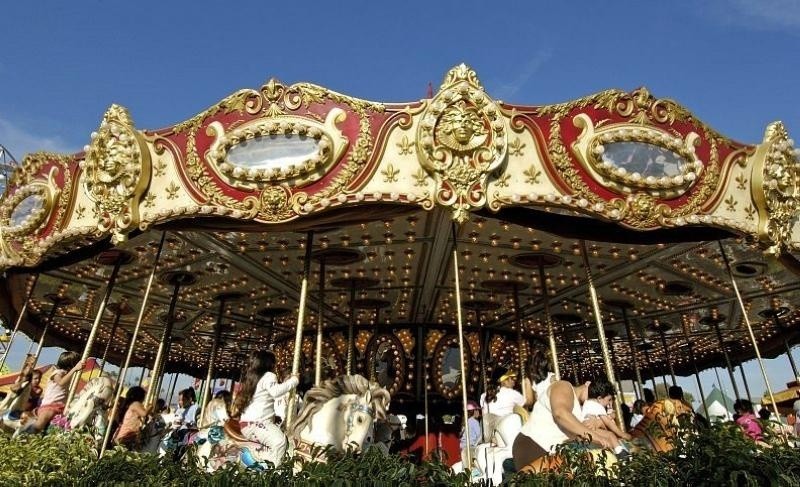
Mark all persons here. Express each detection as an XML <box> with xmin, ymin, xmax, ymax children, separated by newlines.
<box><xmin>447</xmin><ymin>351</ymin><xmax>695</xmax><ymax>487</ymax></box>
<box><xmin>8</xmin><ymin>370</ymin><xmax>43</xmax><ymax>421</ymax></box>
<box><xmin>733</xmin><ymin>399</ymin><xmax>800</xmax><ymax>446</ymax></box>
<box><xmin>115</xmin><ymin>350</ymin><xmax>300</xmax><ymax>467</ymax></box>
<box><xmin>12</xmin><ymin>351</ymin><xmax>86</xmax><ymax>439</ymax></box>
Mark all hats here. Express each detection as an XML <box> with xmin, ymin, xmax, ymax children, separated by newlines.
<box><xmin>497</xmin><ymin>370</ymin><xmax>518</xmax><ymax>383</ymax></box>
<box><xmin>467</xmin><ymin>399</ymin><xmax>481</xmax><ymax>410</ymax></box>
